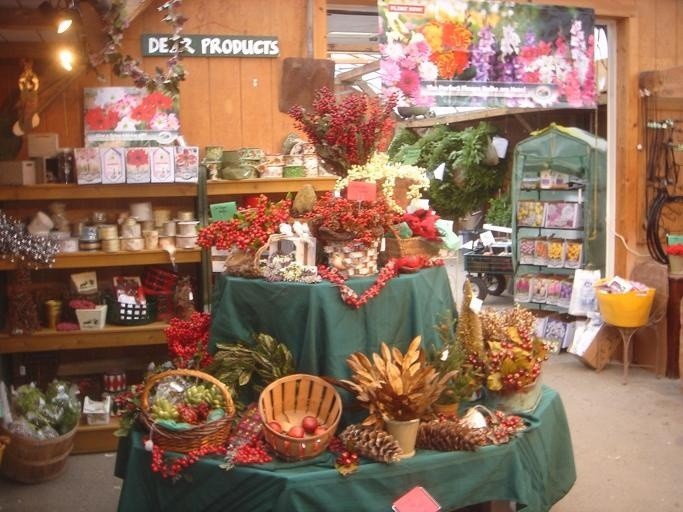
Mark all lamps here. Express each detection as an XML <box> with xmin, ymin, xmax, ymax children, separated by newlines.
<box><xmin>38</xmin><ymin>0</ymin><xmax>73</xmax><ymax>34</ymax></box>
<box><xmin>53</xmin><ymin>8</ymin><xmax>92</xmax><ymax>77</ymax></box>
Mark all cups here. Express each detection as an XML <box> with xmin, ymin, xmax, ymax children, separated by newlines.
<box><xmin>46</xmin><ymin>299</ymin><xmax>63</xmax><ymax>327</ymax></box>
<box><xmin>264</xmin><ymin>152</ymin><xmax>320</xmax><ymax>179</ymax></box>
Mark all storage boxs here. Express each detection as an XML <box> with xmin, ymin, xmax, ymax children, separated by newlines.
<box><xmin>568</xmin><ymin>315</ymin><xmax>624</xmax><ymax>372</ymax></box>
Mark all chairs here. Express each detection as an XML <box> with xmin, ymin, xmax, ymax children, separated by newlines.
<box><xmin>595</xmin><ymin>261</ymin><xmax>669</xmax><ymax>385</ymax></box>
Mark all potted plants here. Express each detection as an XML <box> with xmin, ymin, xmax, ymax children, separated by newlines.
<box><xmin>341</xmin><ymin>334</ymin><xmax>459</xmax><ymax>458</ymax></box>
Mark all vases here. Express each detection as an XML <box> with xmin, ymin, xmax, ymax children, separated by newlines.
<box><xmin>75</xmin><ymin>305</ymin><xmax>108</xmax><ymax>332</ymax></box>
<box><xmin>432</xmin><ymin>376</ymin><xmax>543</xmax><ymax>417</ymax></box>
<box><xmin>69</xmin><ymin>271</ymin><xmax>99</xmax><ymax>295</ymax></box>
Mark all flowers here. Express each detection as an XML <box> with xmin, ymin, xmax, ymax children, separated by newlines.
<box><xmin>162</xmin><ymin>313</ymin><xmax>211</xmax><ymax>366</ymax></box>
<box><xmin>458</xmin><ymin>278</ymin><xmax>553</xmax><ymax>391</ymax></box>
<box><xmin>79</xmin><ymin>86</ymin><xmax>197</xmax><ymax>179</ymax></box>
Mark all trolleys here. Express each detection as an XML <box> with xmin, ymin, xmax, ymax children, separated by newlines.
<box><xmin>464</xmin><ymin>233</ymin><xmax>516</xmax><ymax>300</ymax></box>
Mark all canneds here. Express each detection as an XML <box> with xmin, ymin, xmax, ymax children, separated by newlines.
<box><xmin>203</xmin><ymin>145</ymin><xmax>319</xmax><ymax>181</ymax></box>
<box><xmin>45</xmin><ymin>299</ymin><xmax>62</xmax><ymax>329</ymax></box>
<box><xmin>80</xmin><ymin>202</ymin><xmax>198</xmax><ymax>251</ymax></box>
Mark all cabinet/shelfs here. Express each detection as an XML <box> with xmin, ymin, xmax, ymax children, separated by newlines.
<box><xmin>0</xmin><ymin>181</ymin><xmax>208</xmax><ymax>455</ymax></box>
<box><xmin>114</xmin><ymin>263</ymin><xmax>577</xmax><ymax>512</ymax></box>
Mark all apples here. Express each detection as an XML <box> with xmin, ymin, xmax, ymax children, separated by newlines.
<box><xmin>315</xmin><ymin>425</ymin><xmax>326</xmax><ymax>436</ymax></box>
<box><xmin>267</xmin><ymin>422</ymin><xmax>281</xmax><ymax>433</ymax></box>
<box><xmin>288</xmin><ymin>426</ymin><xmax>305</xmax><ymax>438</ymax></box>
<box><xmin>302</xmin><ymin>416</ymin><xmax>319</xmax><ymax>433</ymax></box>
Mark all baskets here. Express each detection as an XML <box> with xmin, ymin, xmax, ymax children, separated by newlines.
<box><xmin>223</xmin><ymin>249</ymin><xmax>258</xmax><ymax>278</ymax></box>
<box><xmin>0</xmin><ymin>416</ymin><xmax>78</xmax><ymax>486</ymax></box>
<box><xmin>257</xmin><ymin>374</ymin><xmax>343</xmax><ymax>461</ymax></box>
<box><xmin>325</xmin><ymin>233</ymin><xmax>380</xmax><ymax>279</ymax></box>
<box><xmin>102</xmin><ymin>267</ymin><xmax>179</xmax><ymax>326</ymax></box>
<box><xmin>138</xmin><ymin>368</ymin><xmax>236</xmax><ymax>453</ymax></box>
<box><xmin>379</xmin><ymin>234</ymin><xmax>440</xmax><ymax>262</ymax></box>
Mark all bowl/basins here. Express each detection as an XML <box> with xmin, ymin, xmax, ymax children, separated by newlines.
<box><xmin>205</xmin><ymin>146</ymin><xmax>224</xmax><ymax>160</ymax></box>
<box><xmin>592</xmin><ymin>285</ymin><xmax>655</xmax><ymax>327</ymax></box>
